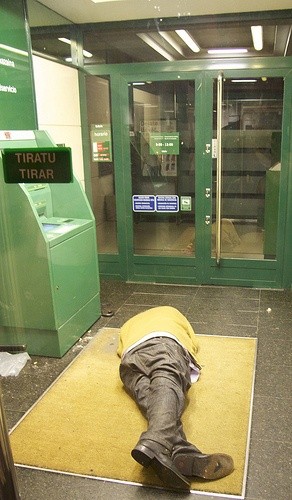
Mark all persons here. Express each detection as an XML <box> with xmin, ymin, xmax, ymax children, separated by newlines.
<box><xmin>116</xmin><ymin>304</ymin><xmax>235</xmax><ymax>492</ymax></box>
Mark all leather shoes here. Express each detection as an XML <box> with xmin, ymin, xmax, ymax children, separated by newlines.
<box><xmin>131</xmin><ymin>439</ymin><xmax>192</xmax><ymax>491</ymax></box>
<box><xmin>173</xmin><ymin>449</ymin><xmax>234</xmax><ymax>479</ymax></box>
